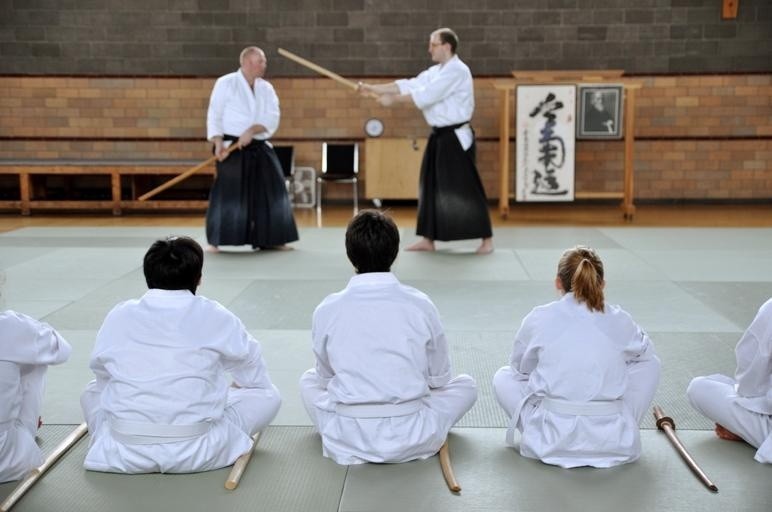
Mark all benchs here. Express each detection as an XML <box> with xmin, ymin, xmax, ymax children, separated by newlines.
<box><xmin>0</xmin><ymin>156</ymin><xmax>217</xmax><ymax>216</ymax></box>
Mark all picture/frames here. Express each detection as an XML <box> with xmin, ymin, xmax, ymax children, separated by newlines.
<box><xmin>576</xmin><ymin>85</ymin><xmax>621</xmax><ymax>138</ymax></box>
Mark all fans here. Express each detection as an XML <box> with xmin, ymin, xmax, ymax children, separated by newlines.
<box><xmin>283</xmin><ymin>167</ymin><xmax>315</xmax><ymax>208</ymax></box>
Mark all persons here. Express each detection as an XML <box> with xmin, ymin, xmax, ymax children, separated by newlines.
<box><xmin>206</xmin><ymin>46</ymin><xmax>298</xmax><ymax>254</ymax></box>
<box><xmin>300</xmin><ymin>208</ymin><xmax>478</xmax><ymax>465</ymax></box>
<box><xmin>492</xmin><ymin>246</ymin><xmax>664</xmax><ymax>468</ymax></box>
<box><xmin>359</xmin><ymin>27</ymin><xmax>493</xmax><ymax>254</ymax></box>
<box><xmin>686</xmin><ymin>297</ymin><xmax>772</xmax><ymax>464</ymax></box>
<box><xmin>0</xmin><ymin>308</ymin><xmax>72</xmax><ymax>483</ymax></box>
<box><xmin>79</xmin><ymin>238</ymin><xmax>282</xmax><ymax>474</ymax></box>
<box><xmin>585</xmin><ymin>92</ymin><xmax>615</xmax><ymax>132</ymax></box>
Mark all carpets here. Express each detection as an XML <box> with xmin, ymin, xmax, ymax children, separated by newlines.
<box><xmin>1</xmin><ymin>225</ymin><xmax>772</xmax><ymax>512</ymax></box>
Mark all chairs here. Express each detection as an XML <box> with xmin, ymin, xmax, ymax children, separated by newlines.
<box><xmin>312</xmin><ymin>141</ymin><xmax>361</xmax><ymax>217</ymax></box>
<box><xmin>272</xmin><ymin>144</ymin><xmax>294</xmax><ymax>211</ymax></box>
